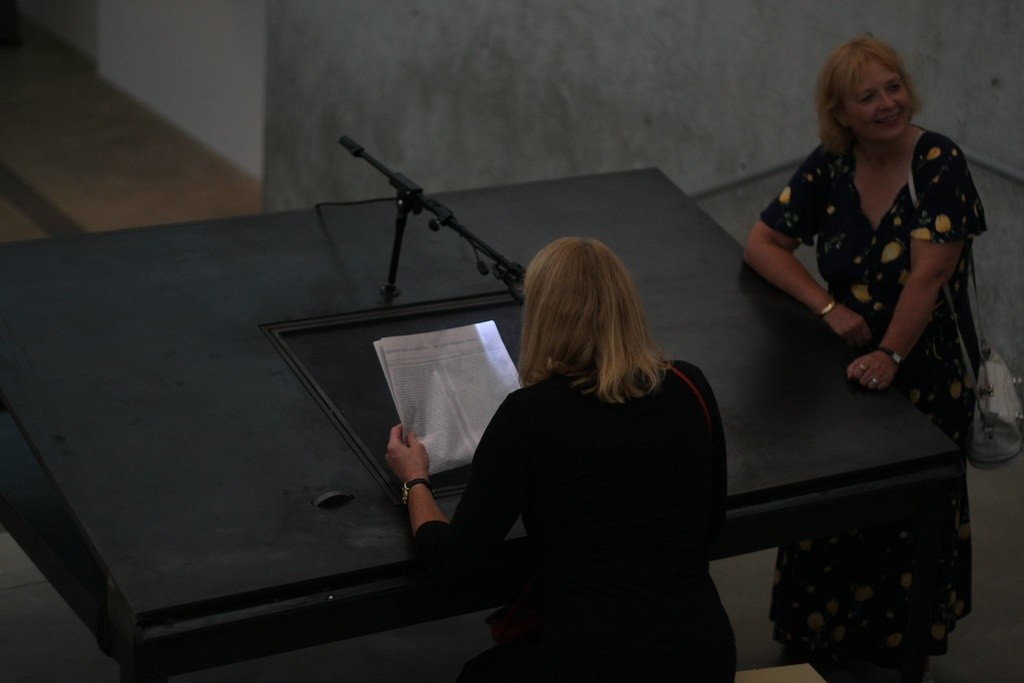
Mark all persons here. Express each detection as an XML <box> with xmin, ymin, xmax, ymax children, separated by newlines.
<box><xmin>745</xmin><ymin>37</ymin><xmax>989</xmax><ymax>683</ymax></box>
<box><xmin>383</xmin><ymin>236</ymin><xmax>735</xmax><ymax>683</ymax></box>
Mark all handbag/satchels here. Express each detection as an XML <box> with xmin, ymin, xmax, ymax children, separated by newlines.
<box><xmin>966</xmin><ymin>338</ymin><xmax>1024</xmax><ymax>471</ymax></box>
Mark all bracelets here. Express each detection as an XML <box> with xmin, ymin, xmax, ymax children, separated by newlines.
<box><xmin>877</xmin><ymin>347</ymin><xmax>902</xmax><ymax>366</ymax></box>
<box><xmin>402</xmin><ymin>478</ymin><xmax>431</xmax><ymax>504</ymax></box>
<box><xmin>821</xmin><ymin>300</ymin><xmax>837</xmax><ymax>315</ymax></box>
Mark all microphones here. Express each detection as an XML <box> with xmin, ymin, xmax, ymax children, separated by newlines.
<box><xmin>496</xmin><ymin>266</ymin><xmax>524</xmax><ymax>305</ymax></box>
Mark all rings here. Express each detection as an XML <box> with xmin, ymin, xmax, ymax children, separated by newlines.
<box><xmin>859</xmin><ymin>365</ymin><xmax>866</xmax><ymax>371</ymax></box>
<box><xmin>873</xmin><ymin>377</ymin><xmax>880</xmax><ymax>383</ymax></box>
